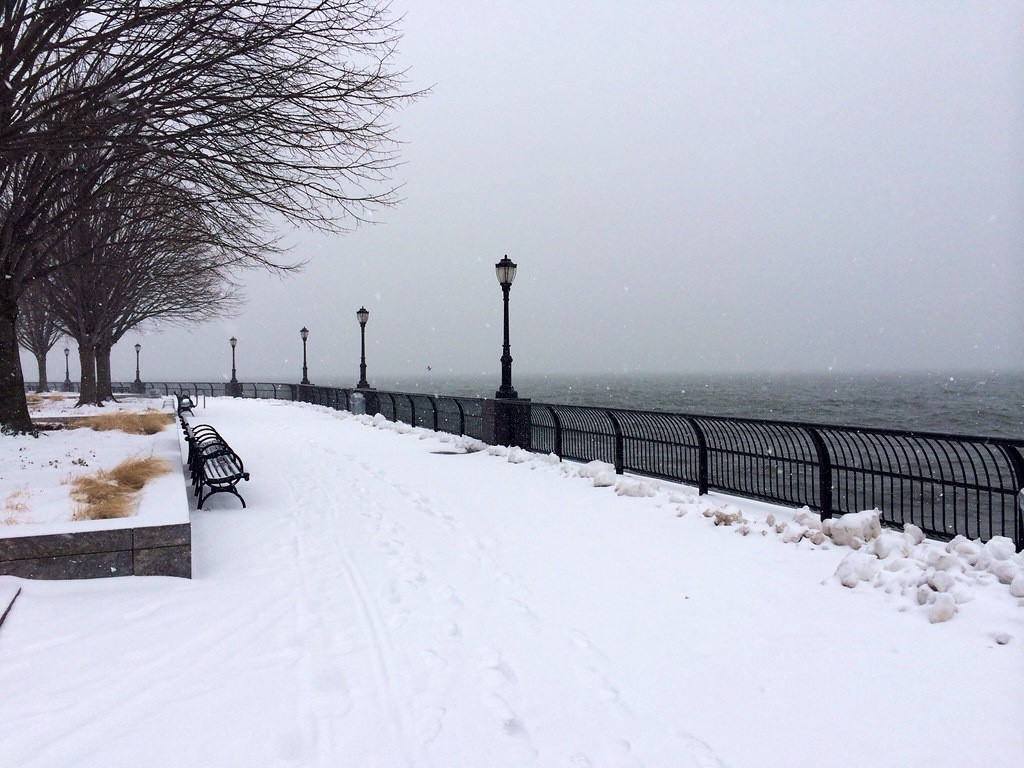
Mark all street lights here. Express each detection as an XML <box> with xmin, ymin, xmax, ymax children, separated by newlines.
<box><xmin>496</xmin><ymin>256</ymin><xmax>519</xmax><ymax>399</ymax></box>
<box><xmin>356</xmin><ymin>306</ymin><xmax>370</xmax><ymax>387</ymax></box>
<box><xmin>135</xmin><ymin>343</ymin><xmax>141</xmax><ymax>383</ymax></box>
<box><xmin>230</xmin><ymin>336</ymin><xmax>238</xmax><ymax>382</ymax></box>
<box><xmin>300</xmin><ymin>327</ymin><xmax>310</xmax><ymax>385</ymax></box>
<box><xmin>64</xmin><ymin>348</ymin><xmax>71</xmax><ymax>383</ymax></box>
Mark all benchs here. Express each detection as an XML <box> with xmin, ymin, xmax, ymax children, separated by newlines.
<box><xmin>173</xmin><ymin>390</ymin><xmax>251</xmax><ymax>510</ymax></box>
